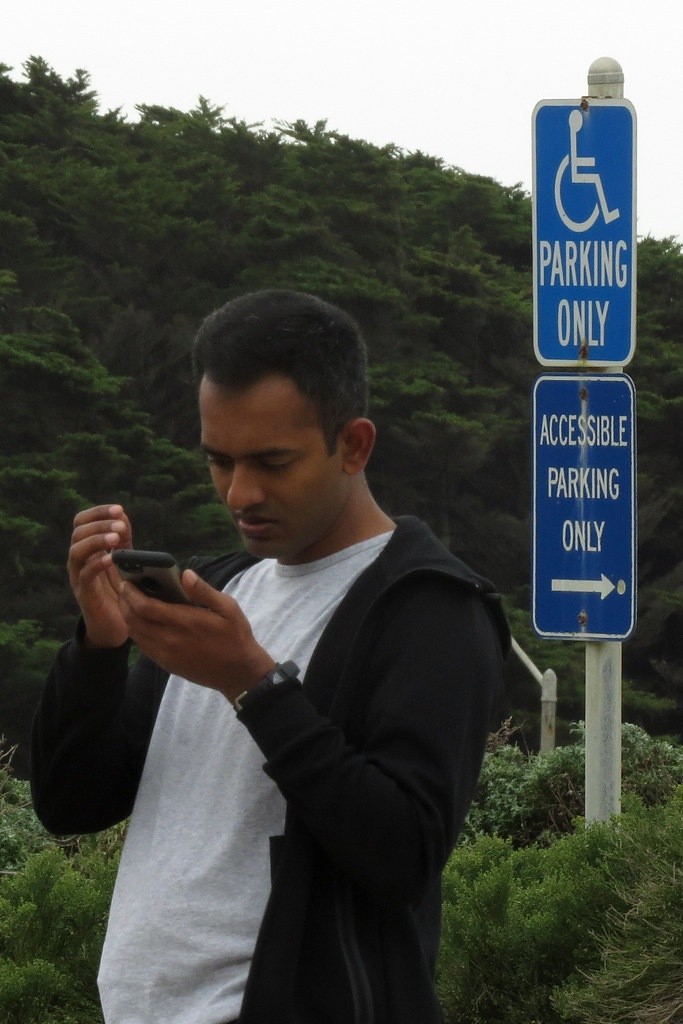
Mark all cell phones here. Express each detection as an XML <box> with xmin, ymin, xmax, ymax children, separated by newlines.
<box><xmin>110</xmin><ymin>550</ymin><xmax>191</xmax><ymax>607</ymax></box>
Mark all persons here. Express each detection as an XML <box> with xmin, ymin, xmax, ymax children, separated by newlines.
<box><xmin>30</xmin><ymin>287</ymin><xmax>510</xmax><ymax>1024</ymax></box>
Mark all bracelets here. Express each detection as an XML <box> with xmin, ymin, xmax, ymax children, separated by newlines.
<box><xmin>233</xmin><ymin>660</ymin><xmax>301</xmax><ymax>713</ymax></box>
